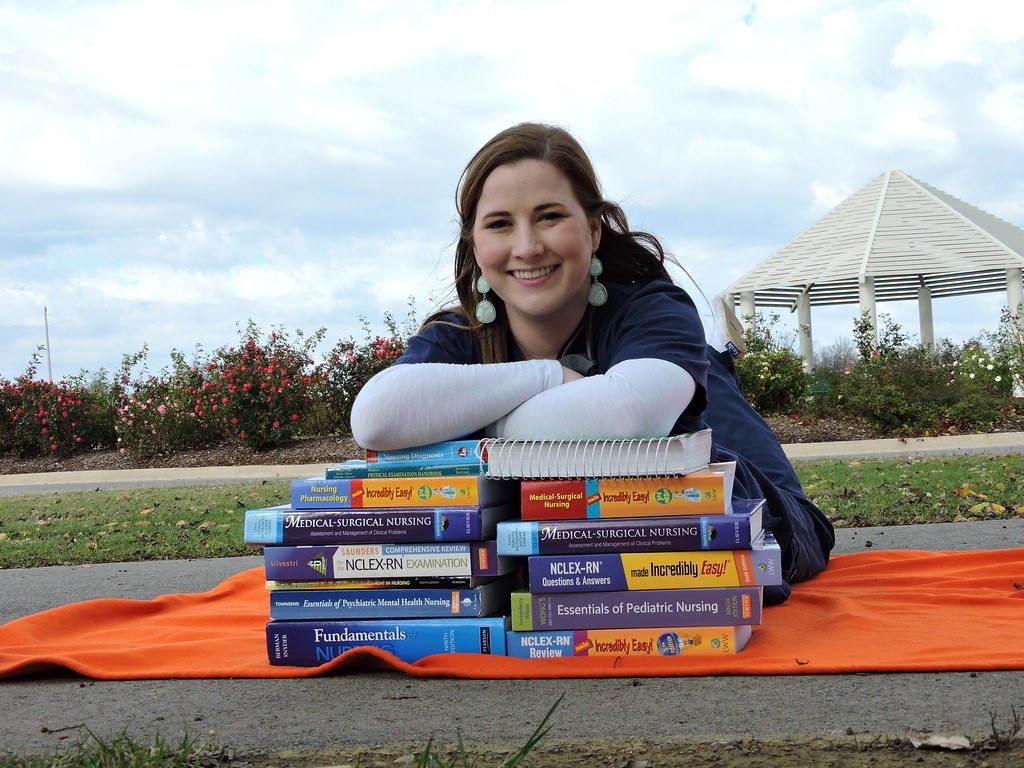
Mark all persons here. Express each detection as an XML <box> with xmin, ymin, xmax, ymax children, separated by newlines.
<box><xmin>348</xmin><ymin>124</ymin><xmax>836</xmax><ymax>606</ymax></box>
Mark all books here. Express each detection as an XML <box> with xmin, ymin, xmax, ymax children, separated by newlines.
<box><xmin>243</xmin><ymin>504</ymin><xmax>518</xmax><ymax>546</ymax></box>
<box><xmin>326</xmin><ymin>459</ymin><xmax>489</xmax><ymax>479</ymax></box>
<box><xmin>366</xmin><ymin>440</ymin><xmax>489</xmax><ymax>468</ymax></box>
<box><xmin>511</xmin><ymin>586</ymin><xmax>765</xmax><ymax>632</ymax></box>
<box><xmin>266</xmin><ymin>606</ymin><xmax>511</xmax><ymax>667</ymax></box>
<box><xmin>290</xmin><ymin>476</ymin><xmax>518</xmax><ymax>509</ymax></box>
<box><xmin>530</xmin><ymin>532</ymin><xmax>782</xmax><ymax>594</ymax></box>
<box><xmin>263</xmin><ymin>538</ymin><xmax>528</xmax><ymax>576</ymax></box>
<box><xmin>496</xmin><ymin>498</ymin><xmax>769</xmax><ymax>557</ymax></box>
<box><xmin>475</xmin><ymin>427</ymin><xmax>712</xmax><ymax>479</ymax></box>
<box><xmin>518</xmin><ymin>461</ymin><xmax>739</xmax><ymax>521</ymax></box>
<box><xmin>269</xmin><ymin>578</ymin><xmax>516</xmax><ymax>621</ymax></box>
<box><xmin>506</xmin><ymin>626</ymin><xmax>753</xmax><ymax>659</ymax></box>
<box><xmin>266</xmin><ymin>577</ymin><xmax>497</xmax><ymax>591</ymax></box>
